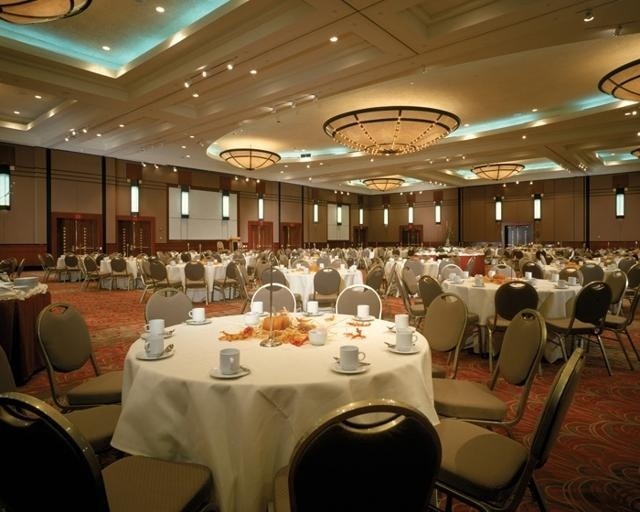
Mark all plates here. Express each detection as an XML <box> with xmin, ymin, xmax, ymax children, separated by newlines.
<box><xmin>136</xmin><ymin>350</ymin><xmax>173</xmax><ymax>361</ymax></box>
<box><xmin>331</xmin><ymin>363</ymin><xmax>366</xmax><ymax>375</ymax></box>
<box><xmin>387</xmin><ymin>345</ymin><xmax>420</xmax><ymax>354</ymax></box>
<box><xmin>183</xmin><ymin>318</ymin><xmax>212</xmax><ymax>325</ymax></box>
<box><xmin>245</xmin><ymin>311</ymin><xmax>271</xmax><ymax>317</ymax></box>
<box><xmin>302</xmin><ymin>311</ymin><xmax>324</xmax><ymax>316</ymax></box>
<box><xmin>140</xmin><ymin>330</ymin><xmax>175</xmax><ymax>339</ymax></box>
<box><xmin>352</xmin><ymin>314</ymin><xmax>375</xmax><ymax>321</ymax></box>
<box><xmin>210</xmin><ymin>366</ymin><xmax>250</xmax><ymax>380</ymax></box>
<box><xmin>14</xmin><ymin>276</ymin><xmax>39</xmax><ymax>290</ymax></box>
<box><xmin>389</xmin><ymin>326</ymin><xmax>416</xmax><ymax>333</ymax></box>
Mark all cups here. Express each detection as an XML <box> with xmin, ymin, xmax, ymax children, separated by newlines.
<box><xmin>340</xmin><ymin>345</ymin><xmax>366</xmax><ymax>370</ymax></box>
<box><xmin>273</xmin><ymin>261</ymin><xmax>356</xmax><ymax>275</ymax></box>
<box><xmin>144</xmin><ymin>318</ymin><xmax>165</xmax><ymax>336</ymax></box>
<box><xmin>219</xmin><ymin>348</ymin><xmax>241</xmax><ymax>375</ymax></box>
<box><xmin>188</xmin><ymin>307</ymin><xmax>205</xmax><ymax>321</ymax></box>
<box><xmin>144</xmin><ymin>336</ymin><xmax>164</xmax><ymax>357</ymax></box>
<box><xmin>240</xmin><ymin>312</ymin><xmax>257</xmax><ymax>324</ymax></box>
<box><xmin>357</xmin><ymin>305</ymin><xmax>370</xmax><ymax>319</ymax></box>
<box><xmin>394</xmin><ymin>313</ymin><xmax>409</xmax><ymax>330</ymax></box>
<box><xmin>396</xmin><ymin>328</ymin><xmax>417</xmax><ymax>351</ymax></box>
<box><xmin>306</xmin><ymin>300</ymin><xmax>319</xmax><ymax>315</ymax></box>
<box><xmin>446</xmin><ymin>269</ymin><xmax>579</xmax><ymax>290</ymax></box>
<box><xmin>251</xmin><ymin>301</ymin><xmax>263</xmax><ymax>314</ymax></box>
<box><xmin>308</xmin><ymin>328</ymin><xmax>326</xmax><ymax>346</ymax></box>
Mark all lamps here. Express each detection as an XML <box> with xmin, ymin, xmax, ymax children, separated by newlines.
<box><xmin>610</xmin><ymin>187</ymin><xmax>629</xmax><ymax>220</ymax></box>
<box><xmin>218</xmin><ymin>149</ymin><xmax>282</xmax><ymax>171</ymax></box>
<box><xmin>321</xmin><ymin>104</ymin><xmax>462</xmax><ymax>161</ymax></box>
<box><xmin>0</xmin><ymin>162</ymin><xmax>12</xmax><ymax>213</ymax></box>
<box><xmin>1</xmin><ymin>0</ymin><xmax>94</xmax><ymax>27</ymax></box>
<box><xmin>598</xmin><ymin>57</ymin><xmax>639</xmax><ymax>102</ymax></box>
<box><xmin>469</xmin><ymin>162</ymin><xmax>526</xmax><ymax>182</ymax></box>
